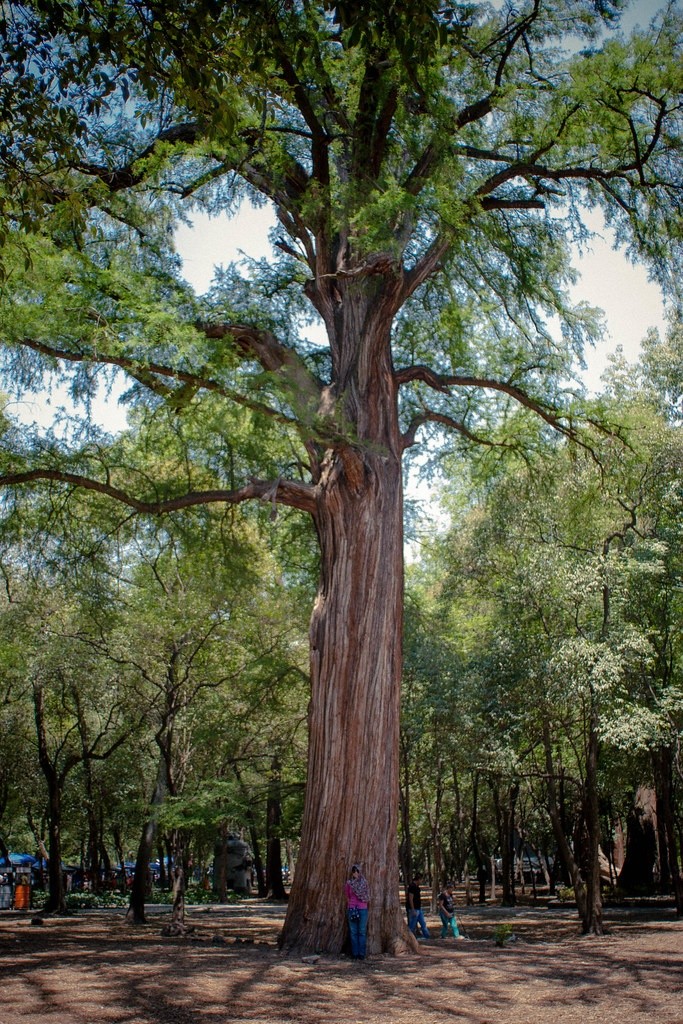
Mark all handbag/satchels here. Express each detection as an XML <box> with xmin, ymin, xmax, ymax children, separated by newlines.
<box><xmin>348</xmin><ymin>906</ymin><xmax>360</xmax><ymax>922</ymax></box>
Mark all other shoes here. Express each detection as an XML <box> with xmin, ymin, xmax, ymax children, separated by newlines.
<box><xmin>353</xmin><ymin>956</ymin><xmax>357</xmax><ymax>960</ymax></box>
<box><xmin>455</xmin><ymin>935</ymin><xmax>464</xmax><ymax>939</ymax></box>
<box><xmin>359</xmin><ymin>955</ymin><xmax>364</xmax><ymax>960</ymax></box>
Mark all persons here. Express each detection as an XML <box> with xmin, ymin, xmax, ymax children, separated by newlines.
<box><xmin>0</xmin><ymin>873</ymin><xmax>72</xmax><ymax>892</ymax></box>
<box><xmin>345</xmin><ymin>865</ymin><xmax>370</xmax><ymax>961</ymax></box>
<box><xmin>407</xmin><ymin>873</ymin><xmax>465</xmax><ymax>941</ymax></box>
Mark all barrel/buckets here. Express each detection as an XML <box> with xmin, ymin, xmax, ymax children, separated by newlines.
<box><xmin>0</xmin><ymin>884</ymin><xmax>11</xmax><ymax>910</ymax></box>
<box><xmin>14</xmin><ymin>885</ymin><xmax>30</xmax><ymax>910</ymax></box>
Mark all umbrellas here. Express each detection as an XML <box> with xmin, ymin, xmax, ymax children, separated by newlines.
<box><xmin>0</xmin><ymin>853</ymin><xmax>174</xmax><ymax>872</ymax></box>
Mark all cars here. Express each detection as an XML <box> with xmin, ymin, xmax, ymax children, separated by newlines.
<box><xmin>475</xmin><ymin>857</ymin><xmax>555</xmax><ymax>886</ymax></box>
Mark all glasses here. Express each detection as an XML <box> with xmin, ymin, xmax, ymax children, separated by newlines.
<box><xmin>352</xmin><ymin>869</ymin><xmax>359</xmax><ymax>873</ymax></box>
